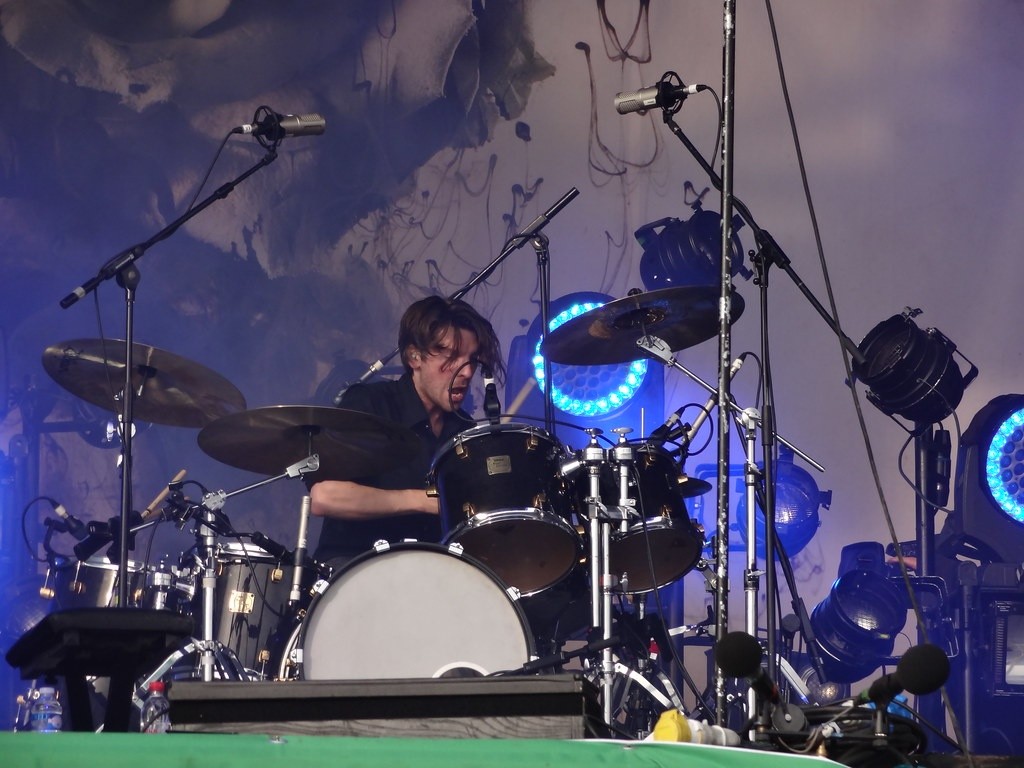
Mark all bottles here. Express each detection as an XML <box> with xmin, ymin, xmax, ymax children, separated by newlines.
<box><xmin>140</xmin><ymin>682</ymin><xmax>170</xmax><ymax>733</ymax></box>
<box><xmin>29</xmin><ymin>687</ymin><xmax>62</xmax><ymax>734</ymax></box>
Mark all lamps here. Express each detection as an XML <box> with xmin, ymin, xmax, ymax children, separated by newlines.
<box><xmin>507</xmin><ymin>205</ymin><xmax>1024</xmax><ymax>750</ymax></box>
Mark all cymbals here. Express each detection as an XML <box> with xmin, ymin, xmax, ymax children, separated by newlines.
<box><xmin>41</xmin><ymin>337</ymin><xmax>247</xmax><ymax>429</ymax></box>
<box><xmin>538</xmin><ymin>283</ymin><xmax>746</xmax><ymax>368</ymax></box>
<box><xmin>196</xmin><ymin>405</ymin><xmax>427</xmax><ymax>486</ymax></box>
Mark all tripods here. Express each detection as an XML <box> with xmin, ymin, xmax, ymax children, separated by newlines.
<box><xmin>96</xmin><ymin>427</ymin><xmax>318</xmax><ymax>734</ymax></box>
<box><xmin>585</xmin><ymin>450</ymin><xmax>691</xmax><ymax>742</ymax></box>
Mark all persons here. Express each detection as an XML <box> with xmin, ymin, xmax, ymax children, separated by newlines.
<box><xmin>889</xmin><ymin>556</ymin><xmax>917</xmax><ymax>571</ymax></box>
<box><xmin>302</xmin><ymin>295</ymin><xmax>509</xmax><ymax>612</ymax></box>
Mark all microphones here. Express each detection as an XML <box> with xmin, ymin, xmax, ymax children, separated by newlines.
<box><xmin>49</xmin><ymin>498</ymin><xmax>87</xmax><ymax>539</ymax></box>
<box><xmin>333</xmin><ymin>390</ymin><xmax>345</xmax><ymax>405</ymax></box>
<box><xmin>233</xmin><ymin>113</ymin><xmax>326</xmax><ymax>140</ymax></box>
<box><xmin>482</xmin><ymin>370</ymin><xmax>502</xmax><ymax>419</ymax></box>
<box><xmin>645</xmin><ymin>407</ymin><xmax>686</xmax><ymax>447</ymax></box>
<box><xmin>852</xmin><ymin>644</ymin><xmax>951</xmax><ymax>706</ymax></box>
<box><xmin>290</xmin><ymin>495</ymin><xmax>312</xmax><ymax>614</ymax></box>
<box><xmin>614</xmin><ymin>84</ymin><xmax>706</xmax><ymax>114</ymax></box>
<box><xmin>717</xmin><ymin>631</ymin><xmax>782</xmax><ymax>709</ymax></box>
<box><xmin>681</xmin><ymin>353</ymin><xmax>750</xmax><ymax>448</ymax></box>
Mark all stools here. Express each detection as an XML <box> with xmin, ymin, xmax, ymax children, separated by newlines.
<box><xmin>5</xmin><ymin>604</ymin><xmax>196</xmax><ymax>731</ymax></box>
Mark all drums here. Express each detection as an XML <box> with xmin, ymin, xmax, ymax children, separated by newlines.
<box><xmin>54</xmin><ymin>556</ymin><xmax>192</xmax><ymax>685</ymax></box>
<box><xmin>276</xmin><ymin>540</ymin><xmax>541</xmax><ymax>682</ymax></box>
<box><xmin>430</xmin><ymin>421</ymin><xmax>582</xmax><ymax>600</ymax></box>
<box><xmin>196</xmin><ymin>541</ymin><xmax>333</xmax><ymax>677</ymax></box>
<box><xmin>585</xmin><ymin>443</ymin><xmax>707</xmax><ymax>599</ymax></box>
